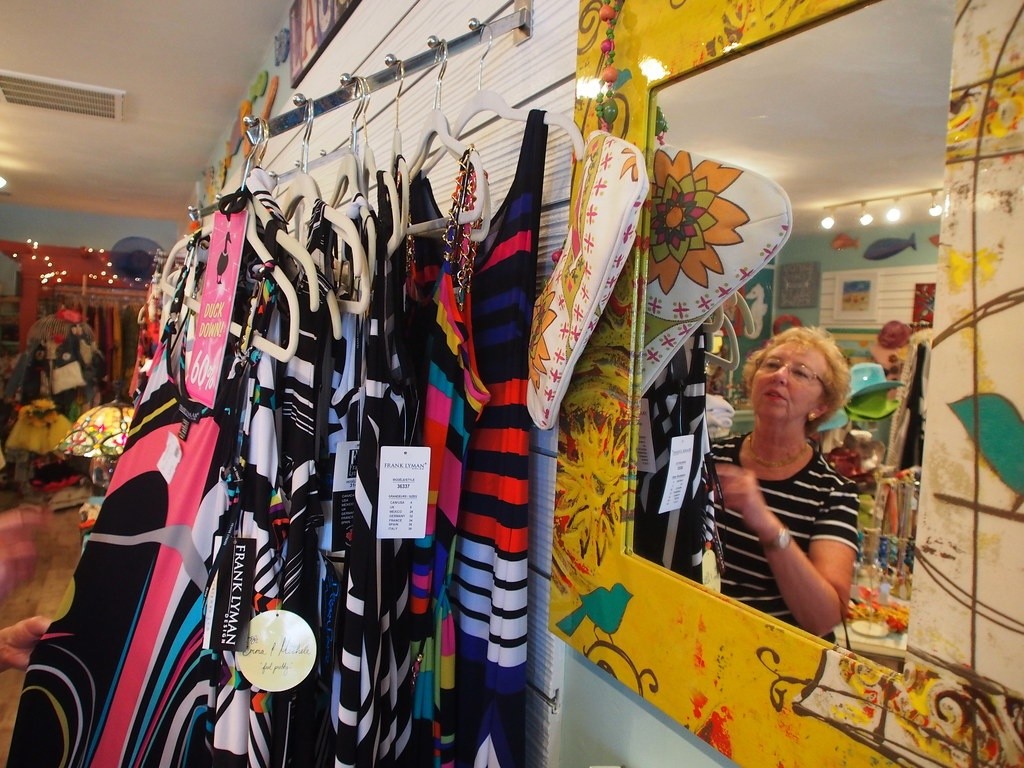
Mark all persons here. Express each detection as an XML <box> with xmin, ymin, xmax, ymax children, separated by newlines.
<box><xmin>705</xmin><ymin>321</ymin><xmax>860</xmax><ymax>639</ymax></box>
<box><xmin>0</xmin><ymin>614</ymin><xmax>56</xmax><ymax>673</ymax></box>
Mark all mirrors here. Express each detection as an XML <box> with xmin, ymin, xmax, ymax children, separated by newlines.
<box><xmin>625</xmin><ymin>0</ymin><xmax>958</xmax><ymax>685</ymax></box>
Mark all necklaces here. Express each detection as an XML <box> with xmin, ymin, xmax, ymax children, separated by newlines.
<box><xmin>747</xmin><ymin>434</ymin><xmax>807</xmax><ymax>468</ymax></box>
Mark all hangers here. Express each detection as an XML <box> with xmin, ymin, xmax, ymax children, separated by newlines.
<box><xmin>158</xmin><ymin>19</ymin><xmax>586</xmax><ymax>364</ymax></box>
<box><xmin>701</xmin><ymin>290</ymin><xmax>757</xmax><ymax>371</ymax></box>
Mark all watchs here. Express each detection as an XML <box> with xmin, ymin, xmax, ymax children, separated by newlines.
<box><xmin>759</xmin><ymin>526</ymin><xmax>791</xmax><ymax>549</ymax></box>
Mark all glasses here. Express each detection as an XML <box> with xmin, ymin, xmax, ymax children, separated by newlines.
<box><xmin>761</xmin><ymin>355</ymin><xmax>829</xmax><ymax>394</ymax></box>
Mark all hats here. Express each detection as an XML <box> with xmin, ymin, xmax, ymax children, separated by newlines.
<box><xmin>815</xmin><ymin>363</ymin><xmax>903</xmax><ymax>432</ymax></box>
